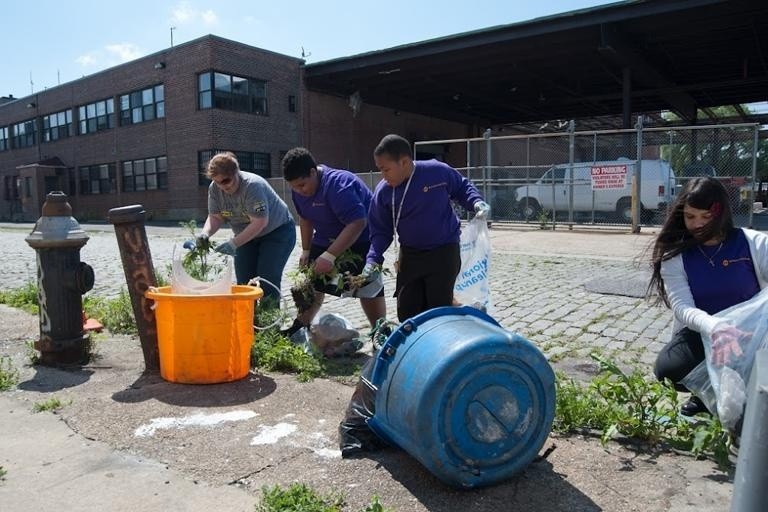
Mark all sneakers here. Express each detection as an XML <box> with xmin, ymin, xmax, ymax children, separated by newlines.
<box><xmin>680</xmin><ymin>394</ymin><xmax>705</xmax><ymax>416</ymax></box>
<box><xmin>276</xmin><ymin>318</ymin><xmax>313</xmax><ymax>343</ymax></box>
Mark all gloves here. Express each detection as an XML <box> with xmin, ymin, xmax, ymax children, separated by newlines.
<box><xmin>361</xmin><ymin>263</ymin><xmax>380</xmax><ymax>282</ymax></box>
<box><xmin>473</xmin><ymin>201</ymin><xmax>491</xmax><ymax>219</ymax></box>
<box><xmin>213</xmin><ymin>240</ymin><xmax>237</xmax><ymax>257</ymax></box>
<box><xmin>181</xmin><ymin>233</ymin><xmax>209</xmax><ymax>249</ymax></box>
<box><xmin>709</xmin><ymin>324</ymin><xmax>754</xmax><ymax>369</ymax></box>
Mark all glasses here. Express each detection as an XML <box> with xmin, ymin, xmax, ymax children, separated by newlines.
<box><xmin>213</xmin><ymin>177</ymin><xmax>233</xmax><ymax>185</ymax></box>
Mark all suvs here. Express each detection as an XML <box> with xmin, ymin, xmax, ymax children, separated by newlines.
<box><xmin>442</xmin><ymin>169</ymin><xmax>511</xmax><ymax>221</ymax></box>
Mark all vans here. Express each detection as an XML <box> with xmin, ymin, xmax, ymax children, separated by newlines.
<box><xmin>511</xmin><ymin>157</ymin><xmax>676</xmax><ymax>222</ymax></box>
<box><xmin>678</xmin><ymin>165</ymin><xmax>716</xmax><ymax>187</ymax></box>
<box><xmin>742</xmin><ymin>181</ymin><xmax>767</xmax><ymax>209</ymax></box>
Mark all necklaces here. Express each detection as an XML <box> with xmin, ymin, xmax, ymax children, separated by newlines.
<box><xmin>695</xmin><ymin>240</ymin><xmax>724</xmax><ymax>269</ymax></box>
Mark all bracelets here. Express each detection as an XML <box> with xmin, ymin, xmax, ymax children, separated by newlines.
<box><xmin>301</xmin><ymin>249</ymin><xmax>309</xmax><ymax>258</ymax></box>
<box><xmin>319</xmin><ymin>251</ymin><xmax>337</xmax><ymax>265</ymax></box>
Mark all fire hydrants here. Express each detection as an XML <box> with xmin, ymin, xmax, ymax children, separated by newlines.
<box><xmin>25</xmin><ymin>189</ymin><xmax>96</xmax><ymax>368</ymax></box>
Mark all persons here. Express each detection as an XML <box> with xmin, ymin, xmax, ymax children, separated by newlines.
<box><xmin>357</xmin><ymin>133</ymin><xmax>492</xmax><ymax>323</ymax></box>
<box><xmin>195</xmin><ymin>151</ymin><xmax>297</xmax><ymax>311</ymax></box>
<box><xmin>636</xmin><ymin>173</ymin><xmax>768</xmax><ymax>442</ymax></box>
<box><xmin>278</xmin><ymin>146</ymin><xmax>392</xmax><ymax>355</ymax></box>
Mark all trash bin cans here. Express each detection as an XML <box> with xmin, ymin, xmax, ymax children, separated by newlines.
<box><xmin>364</xmin><ymin>304</ymin><xmax>556</xmax><ymax>492</ymax></box>
<box><xmin>144</xmin><ymin>285</ymin><xmax>264</xmax><ymax>385</ymax></box>
